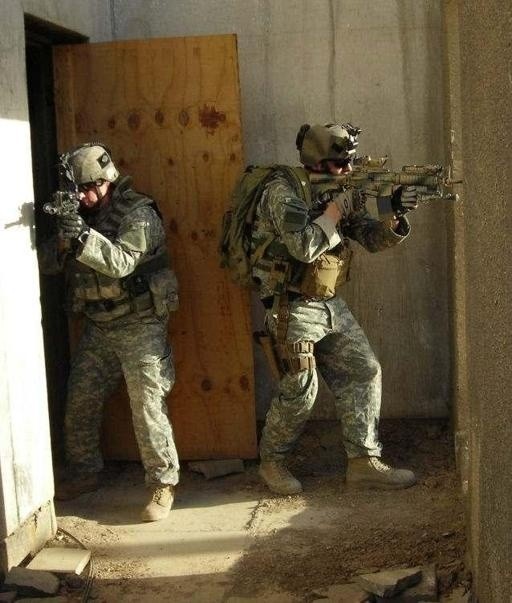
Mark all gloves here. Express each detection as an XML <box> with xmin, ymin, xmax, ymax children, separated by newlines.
<box><xmin>337</xmin><ymin>187</ymin><xmax>368</xmax><ymax>217</ymax></box>
<box><xmin>391</xmin><ymin>184</ymin><xmax>419</xmax><ymax>212</ymax></box>
<box><xmin>59</xmin><ymin>214</ymin><xmax>89</xmax><ymax>239</ymax></box>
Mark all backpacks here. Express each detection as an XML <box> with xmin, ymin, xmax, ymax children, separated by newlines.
<box><xmin>216</xmin><ymin>163</ymin><xmax>313</xmax><ymax>289</ymax></box>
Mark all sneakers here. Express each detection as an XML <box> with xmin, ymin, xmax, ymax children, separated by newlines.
<box><xmin>257</xmin><ymin>460</ymin><xmax>303</xmax><ymax>495</ymax></box>
<box><xmin>140</xmin><ymin>483</ymin><xmax>176</xmax><ymax>520</ymax></box>
<box><xmin>345</xmin><ymin>456</ymin><xmax>417</xmax><ymax>490</ymax></box>
<box><xmin>54</xmin><ymin>471</ymin><xmax>100</xmax><ymax>500</ymax></box>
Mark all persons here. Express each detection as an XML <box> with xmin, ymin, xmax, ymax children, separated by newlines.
<box><xmin>38</xmin><ymin>139</ymin><xmax>181</xmax><ymax>522</ymax></box>
<box><xmin>248</xmin><ymin>121</ymin><xmax>420</xmax><ymax>496</ymax></box>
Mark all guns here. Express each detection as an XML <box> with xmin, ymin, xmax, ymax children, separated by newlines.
<box><xmin>308</xmin><ymin>154</ymin><xmax>463</xmax><ymax>222</ymax></box>
<box><xmin>253</xmin><ymin>329</ymin><xmax>283</xmax><ymax>382</ymax></box>
<box><xmin>43</xmin><ymin>190</ymin><xmax>81</xmax><ymax>254</ymax></box>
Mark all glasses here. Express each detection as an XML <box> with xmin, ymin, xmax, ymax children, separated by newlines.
<box><xmin>77</xmin><ymin>181</ymin><xmax>96</xmax><ymax>192</ymax></box>
<box><xmin>332</xmin><ymin>157</ymin><xmax>350</xmax><ymax>167</ymax></box>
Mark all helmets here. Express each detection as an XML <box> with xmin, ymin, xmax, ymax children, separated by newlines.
<box><xmin>296</xmin><ymin>122</ymin><xmax>362</xmax><ymax>170</ymax></box>
<box><xmin>58</xmin><ymin>144</ymin><xmax>120</xmax><ymax>185</ymax></box>
